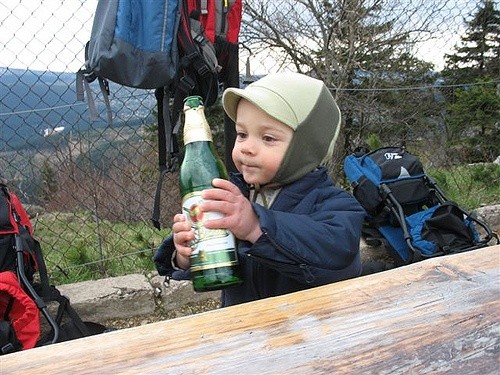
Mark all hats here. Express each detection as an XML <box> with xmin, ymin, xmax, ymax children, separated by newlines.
<box><xmin>222</xmin><ymin>72</ymin><xmax>342</xmax><ymax>208</ymax></box>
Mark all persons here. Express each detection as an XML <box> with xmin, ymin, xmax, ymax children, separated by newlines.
<box><xmin>152</xmin><ymin>70</ymin><xmax>366</xmax><ymax>308</ymax></box>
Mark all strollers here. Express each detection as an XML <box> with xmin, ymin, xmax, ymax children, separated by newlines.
<box><xmin>0</xmin><ymin>184</ymin><xmax>91</xmax><ymax>354</ymax></box>
<box><xmin>343</xmin><ymin>146</ymin><xmax>500</xmax><ymax>268</ymax></box>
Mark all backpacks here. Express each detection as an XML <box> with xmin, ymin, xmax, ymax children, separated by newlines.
<box><xmin>75</xmin><ymin>0</ymin><xmax>183</xmax><ymax>230</ymax></box>
<box><xmin>175</xmin><ymin>0</ymin><xmax>242</xmax><ymax>103</ymax></box>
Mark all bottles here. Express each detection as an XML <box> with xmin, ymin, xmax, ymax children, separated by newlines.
<box><xmin>178</xmin><ymin>96</ymin><xmax>243</xmax><ymax>292</ymax></box>
